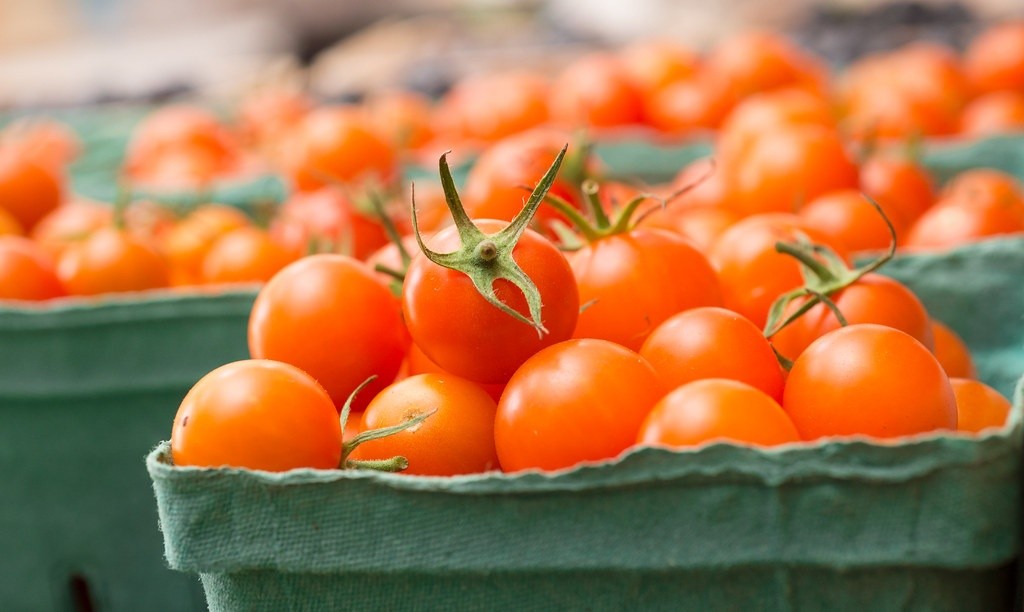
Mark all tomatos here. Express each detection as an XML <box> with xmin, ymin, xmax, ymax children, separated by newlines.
<box><xmin>0</xmin><ymin>19</ymin><xmax>1024</xmax><ymax>477</ymax></box>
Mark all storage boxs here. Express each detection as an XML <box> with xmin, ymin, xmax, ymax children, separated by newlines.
<box><xmin>0</xmin><ymin>98</ymin><xmax>1024</xmax><ymax>612</ymax></box>
